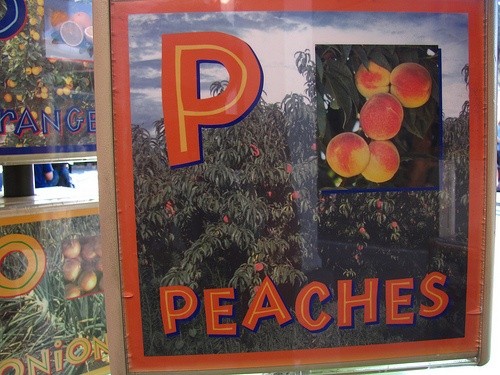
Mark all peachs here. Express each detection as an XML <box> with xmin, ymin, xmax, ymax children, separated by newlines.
<box><xmin>285</xmin><ymin>162</ymin><xmax>293</xmax><ymax>173</ymax></box>
<box><xmin>357</xmin><ymin>245</ymin><xmax>363</xmax><ymax>252</ymax></box>
<box><xmin>392</xmin><ymin>221</ymin><xmax>398</xmax><ymax>227</ymax></box>
<box><xmin>377</xmin><ymin>200</ymin><xmax>383</xmax><ymax>207</ymax></box>
<box><xmin>360</xmin><ymin>92</ymin><xmax>402</xmax><ymax>139</ymax></box>
<box><xmin>254</xmin><ymin>286</ymin><xmax>259</xmax><ymax>293</ymax></box>
<box><xmin>240</xmin><ymin>141</ymin><xmax>261</xmax><ymax>158</ymax></box>
<box><xmin>266</xmin><ymin>191</ymin><xmax>272</xmax><ymax>197</ymax></box>
<box><xmin>310</xmin><ymin>142</ymin><xmax>318</xmax><ymax>151</ymax></box>
<box><xmin>224</xmin><ymin>215</ymin><xmax>230</xmax><ymax>225</ymax></box>
<box><xmin>359</xmin><ymin>140</ymin><xmax>400</xmax><ymax>183</ymax></box>
<box><xmin>254</xmin><ymin>262</ymin><xmax>263</xmax><ymax>272</ymax></box>
<box><xmin>292</xmin><ymin>191</ymin><xmax>300</xmax><ymax>199</ymax></box>
<box><xmin>164</xmin><ymin>201</ymin><xmax>176</xmax><ymax>221</ymax></box>
<box><xmin>354</xmin><ymin>59</ymin><xmax>432</xmax><ymax>108</ymax></box>
<box><xmin>359</xmin><ymin>227</ymin><xmax>366</xmax><ymax>234</ymax></box>
<box><xmin>326</xmin><ymin>131</ymin><xmax>368</xmax><ymax>178</ymax></box>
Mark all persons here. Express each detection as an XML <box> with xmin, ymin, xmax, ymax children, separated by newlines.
<box><xmin>33</xmin><ymin>164</ymin><xmax>75</xmax><ymax>190</ymax></box>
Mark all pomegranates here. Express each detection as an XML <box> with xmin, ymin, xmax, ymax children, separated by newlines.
<box><xmin>61</xmin><ymin>236</ymin><xmax>102</xmax><ymax>299</ymax></box>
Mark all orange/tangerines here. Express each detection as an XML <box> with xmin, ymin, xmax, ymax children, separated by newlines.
<box><xmin>5</xmin><ymin>0</ymin><xmax>91</xmax><ymax>122</ymax></box>
<box><xmin>52</xmin><ymin>9</ymin><xmax>94</xmax><ymax>47</ymax></box>
